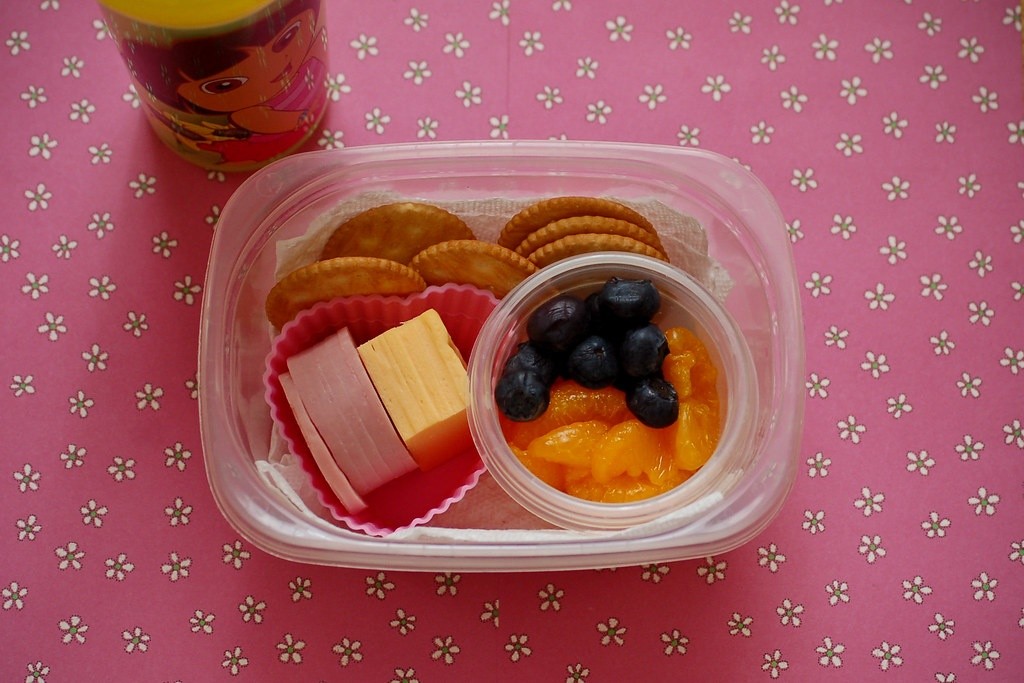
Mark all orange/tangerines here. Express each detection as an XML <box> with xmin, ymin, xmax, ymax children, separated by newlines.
<box><xmin>505</xmin><ymin>324</ymin><xmax>721</xmax><ymax>503</ymax></box>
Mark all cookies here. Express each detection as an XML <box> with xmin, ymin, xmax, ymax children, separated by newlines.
<box><xmin>263</xmin><ymin>195</ymin><xmax>669</xmax><ymax>327</ymax></box>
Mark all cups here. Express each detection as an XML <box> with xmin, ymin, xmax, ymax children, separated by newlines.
<box><xmin>94</xmin><ymin>1</ymin><xmax>332</xmax><ymax>173</ymax></box>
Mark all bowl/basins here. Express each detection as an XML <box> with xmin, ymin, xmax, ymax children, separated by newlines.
<box><xmin>463</xmin><ymin>250</ymin><xmax>761</xmax><ymax>538</ymax></box>
<box><xmin>198</xmin><ymin>136</ymin><xmax>807</xmax><ymax>575</ymax></box>
